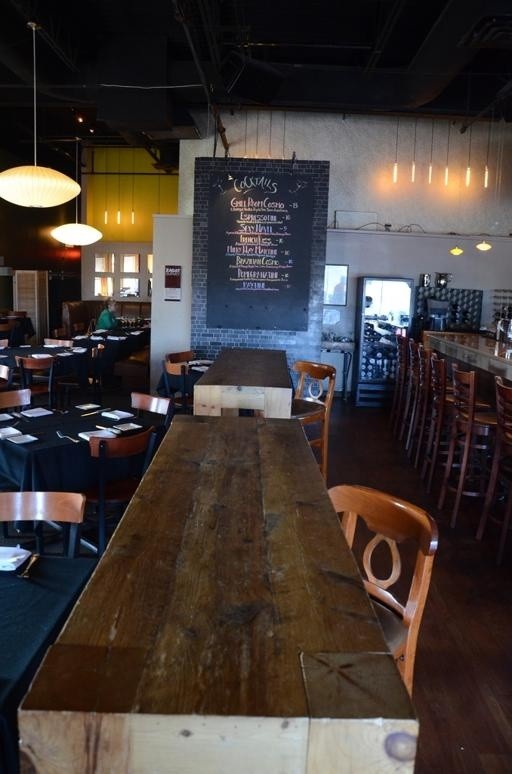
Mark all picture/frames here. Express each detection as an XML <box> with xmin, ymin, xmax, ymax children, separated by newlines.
<box><xmin>322</xmin><ymin>264</ymin><xmax>349</xmax><ymax>306</ymax></box>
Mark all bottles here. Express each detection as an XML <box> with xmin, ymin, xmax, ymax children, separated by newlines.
<box><xmin>361</xmin><ymin>320</ymin><xmax>399</xmax><ymax>381</ymax></box>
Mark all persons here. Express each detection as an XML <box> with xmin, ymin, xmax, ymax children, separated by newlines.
<box><xmin>93</xmin><ymin>298</ymin><xmax>121</xmax><ymax>331</ymax></box>
<box><xmin>365</xmin><ymin>296</ymin><xmax>373</xmax><ymax>307</ymax></box>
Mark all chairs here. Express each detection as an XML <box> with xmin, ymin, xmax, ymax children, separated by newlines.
<box><xmin>326</xmin><ymin>481</ymin><xmax>438</xmax><ymax>703</ymax></box>
<box><xmin>392</xmin><ymin>326</ymin><xmax>512</xmax><ymax>543</ymax></box>
<box><xmin>289</xmin><ymin>360</ymin><xmax>336</xmax><ymax>484</ymax></box>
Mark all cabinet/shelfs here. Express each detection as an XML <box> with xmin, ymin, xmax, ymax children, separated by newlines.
<box><xmin>321</xmin><ymin>352</ymin><xmax>353</xmax><ymax>399</ymax></box>
<box><xmin>417</xmin><ymin>284</ymin><xmax>483</xmax><ymax>333</ymax></box>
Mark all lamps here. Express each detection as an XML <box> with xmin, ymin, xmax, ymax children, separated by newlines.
<box><xmin>448</xmin><ymin>245</ymin><xmax>463</xmax><ymax>256</ymax></box>
<box><xmin>475</xmin><ymin>240</ymin><xmax>492</xmax><ymax>251</ymax></box>
<box><xmin>50</xmin><ymin>138</ymin><xmax>103</xmax><ymax>247</ymax></box>
<box><xmin>223</xmin><ymin>152</ymin><xmax>234</xmax><ymax>181</ymax></box>
<box><xmin>0</xmin><ymin>22</ymin><xmax>83</xmax><ymax>207</ymax></box>
<box><xmin>102</xmin><ymin>148</ymin><xmax>137</xmax><ymax>225</ymax></box>
<box><xmin>288</xmin><ymin>152</ymin><xmax>299</xmax><ymax>178</ymax></box>
<box><xmin>390</xmin><ymin>116</ymin><xmax>495</xmax><ymax>190</ymax></box>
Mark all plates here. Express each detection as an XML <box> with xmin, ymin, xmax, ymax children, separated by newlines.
<box><xmin>5</xmin><ymin>316</ymin><xmax>18</xmax><ymax>319</ymax></box>
<box><xmin>1</xmin><ymin>403</ymin><xmax>144</xmax><ymax>445</ymax></box>
<box><xmin>0</xmin><ymin>344</ymin><xmax>88</xmax><ymax>361</ymax></box>
<box><xmin>1</xmin><ymin>544</ymin><xmax>32</xmax><ymax>571</ymax></box>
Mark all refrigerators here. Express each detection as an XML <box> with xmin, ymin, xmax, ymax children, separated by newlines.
<box><xmin>351</xmin><ymin>276</ymin><xmax>415</xmax><ymax>407</ymax></box>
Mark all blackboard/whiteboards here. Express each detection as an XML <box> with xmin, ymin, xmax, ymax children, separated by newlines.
<box><xmin>206</xmin><ymin>172</ymin><xmax>314</xmax><ymax>331</ymax></box>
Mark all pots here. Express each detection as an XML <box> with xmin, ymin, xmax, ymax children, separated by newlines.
<box><xmin>429</xmin><ymin>312</ymin><xmax>448</xmax><ymax>331</ymax></box>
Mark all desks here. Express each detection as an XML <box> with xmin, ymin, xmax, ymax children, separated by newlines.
<box><xmin>15</xmin><ymin>414</ymin><xmax>421</xmax><ymax>772</ymax></box>
<box><xmin>192</xmin><ymin>345</ymin><xmax>293</xmax><ymax>418</ymax></box>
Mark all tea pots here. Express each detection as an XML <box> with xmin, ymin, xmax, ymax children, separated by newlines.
<box><xmin>434</xmin><ymin>271</ymin><xmax>453</xmax><ymax>287</ymax></box>
